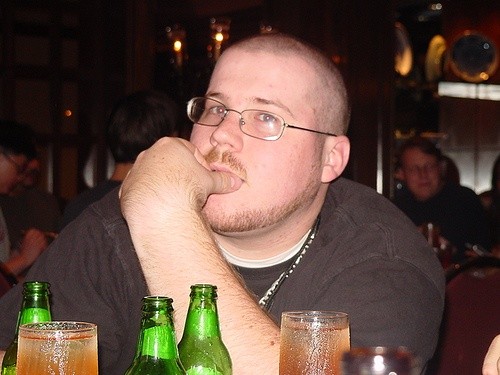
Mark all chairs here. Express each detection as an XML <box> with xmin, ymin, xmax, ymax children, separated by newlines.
<box><xmin>425</xmin><ymin>253</ymin><xmax>500</xmax><ymax>375</ymax></box>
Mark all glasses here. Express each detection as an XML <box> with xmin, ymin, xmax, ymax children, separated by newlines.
<box><xmin>0</xmin><ymin>150</ymin><xmax>26</xmax><ymax>176</ymax></box>
<box><xmin>186</xmin><ymin>96</ymin><xmax>339</xmax><ymax>141</ymax></box>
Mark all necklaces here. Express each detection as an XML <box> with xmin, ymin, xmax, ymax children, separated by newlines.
<box><xmin>261</xmin><ymin>215</ymin><xmax>320</xmax><ymax>314</ymax></box>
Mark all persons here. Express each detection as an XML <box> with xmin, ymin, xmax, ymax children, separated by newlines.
<box><xmin>0</xmin><ymin>94</ymin><xmax>178</xmax><ymax>295</ymax></box>
<box><xmin>0</xmin><ymin>32</ymin><xmax>446</xmax><ymax>375</ymax></box>
<box><xmin>391</xmin><ymin>138</ymin><xmax>500</xmax><ymax>375</ymax></box>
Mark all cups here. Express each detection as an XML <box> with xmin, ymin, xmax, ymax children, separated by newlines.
<box><xmin>423</xmin><ymin>223</ymin><xmax>440</xmax><ymax>249</ymax></box>
<box><xmin>342</xmin><ymin>346</ymin><xmax>414</xmax><ymax>375</ymax></box>
<box><xmin>279</xmin><ymin>309</ymin><xmax>351</xmax><ymax>375</ymax></box>
<box><xmin>16</xmin><ymin>321</ymin><xmax>98</xmax><ymax>375</ymax></box>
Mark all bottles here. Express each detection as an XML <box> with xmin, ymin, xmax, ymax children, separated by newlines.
<box><xmin>1</xmin><ymin>281</ymin><xmax>52</xmax><ymax>375</ymax></box>
<box><xmin>177</xmin><ymin>284</ymin><xmax>232</xmax><ymax>375</ymax></box>
<box><xmin>124</xmin><ymin>296</ymin><xmax>188</xmax><ymax>375</ymax></box>
